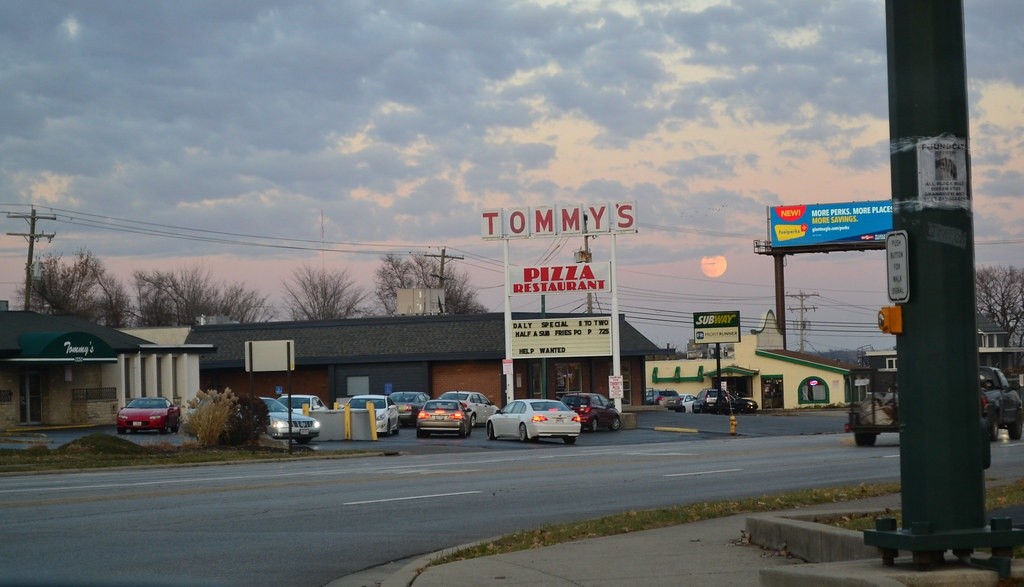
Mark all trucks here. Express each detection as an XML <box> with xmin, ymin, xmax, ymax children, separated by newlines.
<box><xmin>845</xmin><ymin>368</ymin><xmax>900</xmax><ymax>447</ymax></box>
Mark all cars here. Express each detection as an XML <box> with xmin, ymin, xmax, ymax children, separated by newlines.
<box><xmin>238</xmin><ymin>396</ymin><xmax>320</xmax><ymax>445</ymax></box>
<box><xmin>727</xmin><ymin>391</ymin><xmax>758</xmax><ymax>414</ymax></box>
<box><xmin>340</xmin><ymin>394</ymin><xmax>400</xmax><ymax>436</ymax></box>
<box><xmin>115</xmin><ymin>396</ymin><xmax>181</xmax><ymax>435</ymax></box>
<box><xmin>677</xmin><ymin>394</ymin><xmax>699</xmax><ymax>414</ymax></box>
<box><xmin>416</xmin><ymin>399</ymin><xmax>472</xmax><ymax>439</ymax></box>
<box><xmin>485</xmin><ymin>399</ymin><xmax>581</xmax><ymax>446</ymax></box>
<box><xmin>389</xmin><ymin>391</ymin><xmax>430</xmax><ymax>426</ymax></box>
<box><xmin>437</xmin><ymin>389</ymin><xmax>500</xmax><ymax>428</ymax></box>
<box><xmin>560</xmin><ymin>392</ymin><xmax>622</xmax><ymax>433</ymax></box>
<box><xmin>978</xmin><ymin>366</ymin><xmax>1023</xmax><ymax>442</ymax></box>
<box><xmin>275</xmin><ymin>394</ymin><xmax>329</xmax><ymax>419</ymax></box>
<box><xmin>645</xmin><ymin>389</ymin><xmax>682</xmax><ymax>410</ymax></box>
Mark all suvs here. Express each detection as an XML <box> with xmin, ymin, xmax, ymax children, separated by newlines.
<box><xmin>692</xmin><ymin>388</ymin><xmax>731</xmax><ymax>415</ymax></box>
